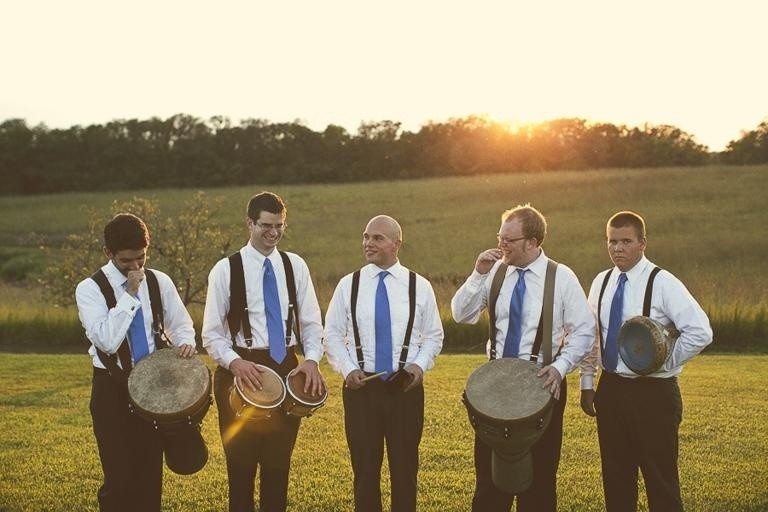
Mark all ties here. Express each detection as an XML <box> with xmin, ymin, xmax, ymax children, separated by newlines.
<box><xmin>125</xmin><ymin>282</ymin><xmax>149</xmax><ymax>362</ymax></box>
<box><xmin>374</xmin><ymin>272</ymin><xmax>393</xmax><ymax>381</ymax></box>
<box><xmin>604</xmin><ymin>275</ymin><xmax>628</xmax><ymax>374</ymax></box>
<box><xmin>263</xmin><ymin>259</ymin><xmax>287</xmax><ymax>364</ymax></box>
<box><xmin>503</xmin><ymin>270</ymin><xmax>525</xmax><ymax>358</ymax></box>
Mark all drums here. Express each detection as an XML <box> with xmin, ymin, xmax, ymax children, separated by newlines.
<box><xmin>128</xmin><ymin>348</ymin><xmax>212</xmax><ymax>474</ymax></box>
<box><xmin>463</xmin><ymin>358</ymin><xmax>554</xmax><ymax>495</ymax></box>
<box><xmin>229</xmin><ymin>365</ymin><xmax>285</xmax><ymax>420</ymax></box>
<box><xmin>284</xmin><ymin>369</ymin><xmax>327</xmax><ymax>418</ymax></box>
<box><xmin>619</xmin><ymin>317</ymin><xmax>679</xmax><ymax>374</ymax></box>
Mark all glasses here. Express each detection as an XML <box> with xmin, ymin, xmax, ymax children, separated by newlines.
<box><xmin>257</xmin><ymin>223</ymin><xmax>288</xmax><ymax>231</ymax></box>
<box><xmin>496</xmin><ymin>233</ymin><xmax>525</xmax><ymax>245</ymax></box>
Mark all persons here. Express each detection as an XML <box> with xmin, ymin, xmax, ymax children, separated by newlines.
<box><xmin>324</xmin><ymin>215</ymin><xmax>444</xmax><ymax>512</ymax></box>
<box><xmin>75</xmin><ymin>214</ymin><xmax>197</xmax><ymax>512</ymax></box>
<box><xmin>580</xmin><ymin>210</ymin><xmax>713</xmax><ymax>512</ymax></box>
<box><xmin>451</xmin><ymin>205</ymin><xmax>597</xmax><ymax>512</ymax></box>
<box><xmin>202</xmin><ymin>191</ymin><xmax>325</xmax><ymax>512</ymax></box>
